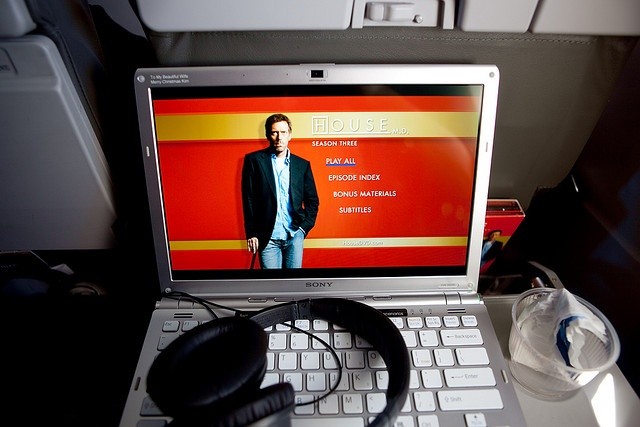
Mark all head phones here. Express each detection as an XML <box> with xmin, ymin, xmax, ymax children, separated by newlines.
<box><xmin>146</xmin><ymin>297</ymin><xmax>409</xmax><ymax>427</ymax></box>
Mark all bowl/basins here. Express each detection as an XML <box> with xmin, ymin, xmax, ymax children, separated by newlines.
<box><xmin>508</xmin><ymin>287</ymin><xmax>622</xmax><ymax>400</ymax></box>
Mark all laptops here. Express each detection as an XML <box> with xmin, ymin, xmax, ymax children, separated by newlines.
<box><xmin>117</xmin><ymin>63</ymin><xmax>530</xmax><ymax>427</ymax></box>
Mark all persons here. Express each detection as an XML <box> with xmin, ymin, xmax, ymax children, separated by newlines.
<box><xmin>240</xmin><ymin>111</ymin><xmax>320</xmax><ymax>268</ymax></box>
